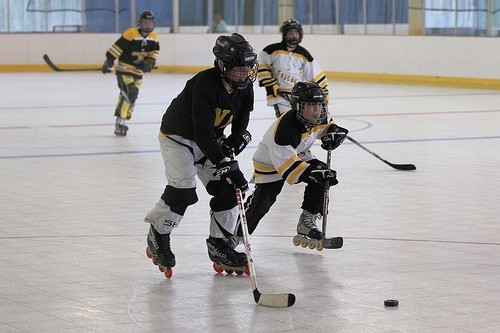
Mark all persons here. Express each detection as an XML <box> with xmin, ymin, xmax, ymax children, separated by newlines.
<box><xmin>146</xmin><ymin>34</ymin><xmax>259</xmax><ymax>279</ymax></box>
<box><xmin>102</xmin><ymin>10</ymin><xmax>160</xmax><ymax>136</ymax></box>
<box><xmin>258</xmin><ymin>19</ymin><xmax>329</xmax><ymax>117</ymax></box>
<box><xmin>237</xmin><ymin>81</ymin><xmax>350</xmax><ymax>252</ymax></box>
<box><xmin>206</xmin><ymin>14</ymin><xmax>227</xmax><ymax>33</ymax></box>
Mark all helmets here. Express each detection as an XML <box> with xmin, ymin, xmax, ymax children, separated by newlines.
<box><xmin>279</xmin><ymin>19</ymin><xmax>304</xmax><ymax>44</ymax></box>
<box><xmin>212</xmin><ymin>33</ymin><xmax>259</xmax><ymax>89</ymax></box>
<box><xmin>137</xmin><ymin>10</ymin><xmax>156</xmax><ymax>32</ymax></box>
<box><xmin>290</xmin><ymin>81</ymin><xmax>328</xmax><ymax>127</ymax></box>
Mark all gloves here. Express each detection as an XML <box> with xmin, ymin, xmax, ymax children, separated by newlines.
<box><xmin>321</xmin><ymin>124</ymin><xmax>348</xmax><ymax>149</ymax></box>
<box><xmin>213</xmin><ymin>161</ymin><xmax>249</xmax><ymax>198</ymax></box>
<box><xmin>225</xmin><ymin>127</ymin><xmax>252</xmax><ymax>156</ymax></box>
<box><xmin>307</xmin><ymin>162</ymin><xmax>338</xmax><ymax>188</ymax></box>
<box><xmin>141</xmin><ymin>58</ymin><xmax>157</xmax><ymax>72</ymax></box>
<box><xmin>102</xmin><ymin>58</ymin><xmax>113</xmax><ymax>74</ymax></box>
<box><xmin>266</xmin><ymin>84</ymin><xmax>279</xmax><ymax>97</ymax></box>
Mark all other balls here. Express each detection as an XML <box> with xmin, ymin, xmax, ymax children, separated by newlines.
<box><xmin>383</xmin><ymin>300</ymin><xmax>399</xmax><ymax>307</ymax></box>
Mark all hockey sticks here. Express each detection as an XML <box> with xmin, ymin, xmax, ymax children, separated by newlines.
<box><xmin>332</xmin><ymin>120</ymin><xmax>418</xmax><ymax>170</ymax></box>
<box><xmin>320</xmin><ymin>145</ymin><xmax>344</xmax><ymax>249</ymax></box>
<box><xmin>227</xmin><ymin>145</ymin><xmax>295</xmax><ymax>310</ymax></box>
<box><xmin>43</xmin><ymin>54</ymin><xmax>159</xmax><ymax>77</ymax></box>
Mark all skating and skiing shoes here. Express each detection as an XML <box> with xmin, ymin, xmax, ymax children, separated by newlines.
<box><xmin>293</xmin><ymin>210</ymin><xmax>325</xmax><ymax>249</ymax></box>
<box><xmin>115</xmin><ymin>117</ymin><xmax>128</xmax><ymax>135</ymax></box>
<box><xmin>147</xmin><ymin>224</ymin><xmax>176</xmax><ymax>279</ymax></box>
<box><xmin>206</xmin><ymin>235</ymin><xmax>250</xmax><ymax>276</ymax></box>
